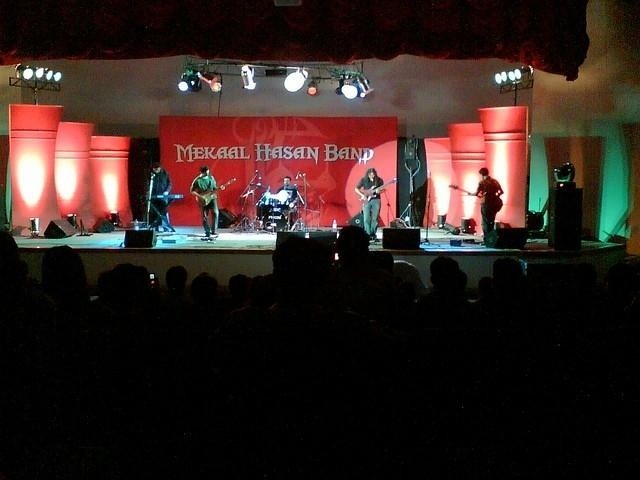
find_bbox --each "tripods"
[396,161,422,227]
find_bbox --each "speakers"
[92,217,113,233]
[43,219,76,238]
[549,187,583,251]
[484,227,529,249]
[382,227,421,250]
[218,208,238,229]
[349,212,364,229]
[125,229,157,248]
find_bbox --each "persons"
[0,221,640,480]
[141,162,173,234]
[189,165,225,241]
[474,168,504,247]
[274,175,299,232]
[353,168,384,242]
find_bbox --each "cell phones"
[149,273,155,285]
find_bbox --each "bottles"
[134,218,139,231]
[332,220,337,234]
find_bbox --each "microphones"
[296,171,299,180]
[151,173,155,178]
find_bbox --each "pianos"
[145,194,184,200]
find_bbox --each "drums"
[277,199,289,207]
[263,212,287,233]
[256,203,273,221]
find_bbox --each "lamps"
[8,63,64,93]
[494,65,535,94]
[493,221,500,230]
[176,57,372,102]
[110,210,121,229]
[30,217,40,239]
[67,212,77,232]
[437,213,446,229]
[462,217,471,234]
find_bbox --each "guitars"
[361,179,397,201]
[196,178,236,205]
[449,185,482,198]
